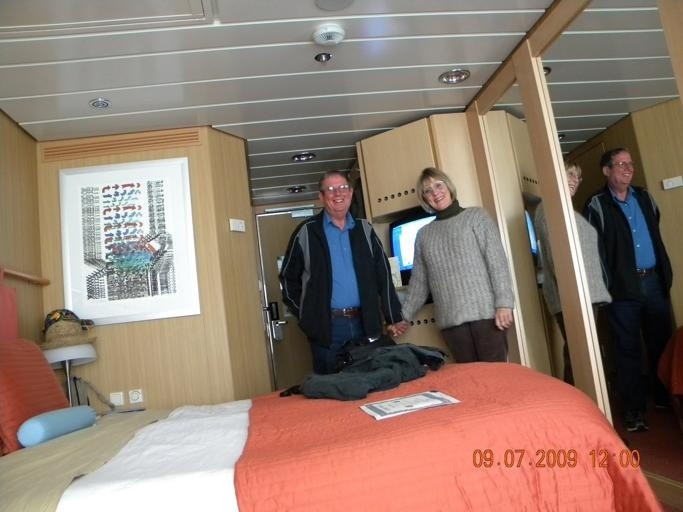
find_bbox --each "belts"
[638,267,657,275]
[330,308,360,317]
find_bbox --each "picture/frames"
[57,157,201,329]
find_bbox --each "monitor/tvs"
[387,212,436,287]
[524,206,540,254]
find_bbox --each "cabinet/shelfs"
[356,112,482,225]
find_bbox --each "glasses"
[609,161,632,169]
[321,184,350,193]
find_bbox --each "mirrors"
[481,2,681,511]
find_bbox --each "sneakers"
[626,416,648,433]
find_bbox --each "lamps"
[44,343,98,406]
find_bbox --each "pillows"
[1,338,96,461]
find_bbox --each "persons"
[582,148,676,432]
[279,170,409,375]
[393,168,515,363]
[533,159,612,386]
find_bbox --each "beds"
[1,359,621,512]
